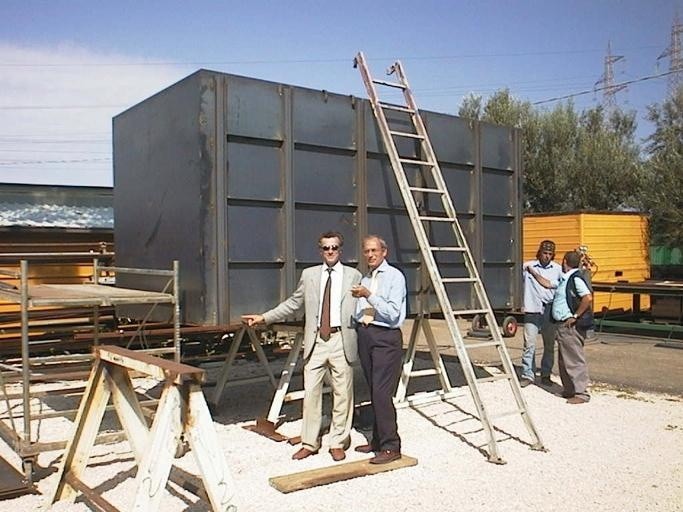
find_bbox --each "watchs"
[573,313,580,319]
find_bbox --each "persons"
[348,234,407,464]
[519,239,563,388]
[240,228,363,460]
[522,251,595,404]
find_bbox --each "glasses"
[363,248,385,256]
[323,245,338,250]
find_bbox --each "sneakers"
[521,379,531,387]
[542,376,553,386]
[567,398,585,404]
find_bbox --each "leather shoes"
[292,448,318,459]
[329,449,346,461]
[355,445,380,453]
[369,450,401,464]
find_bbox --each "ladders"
[353,53,546,465]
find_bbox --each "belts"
[331,326,341,333]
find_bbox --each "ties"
[363,270,378,325]
[320,268,333,342]
